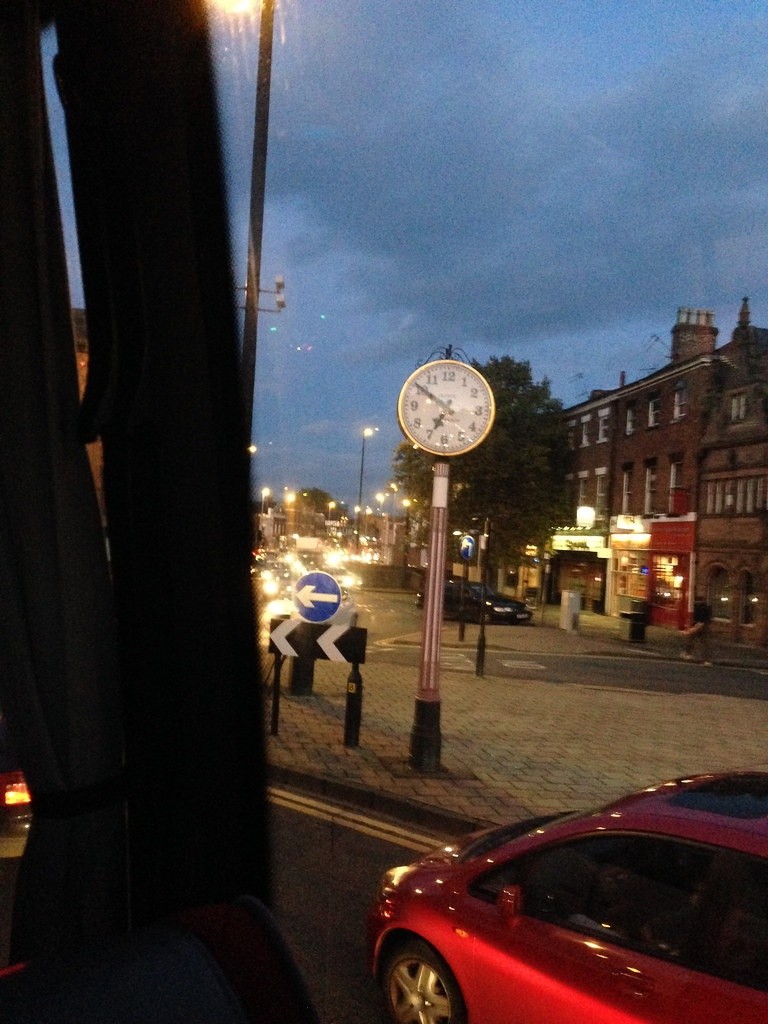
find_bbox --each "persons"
[678,597,713,666]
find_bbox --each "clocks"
[396,359,496,461]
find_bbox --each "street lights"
[353,426,376,554]
[375,492,386,542]
[390,483,399,549]
[365,505,373,541]
[259,486,270,549]
[327,501,337,540]
[403,497,412,589]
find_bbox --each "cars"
[372,766,768,1024]
[415,579,535,624]
[0,753,32,866]
[251,536,381,600]
